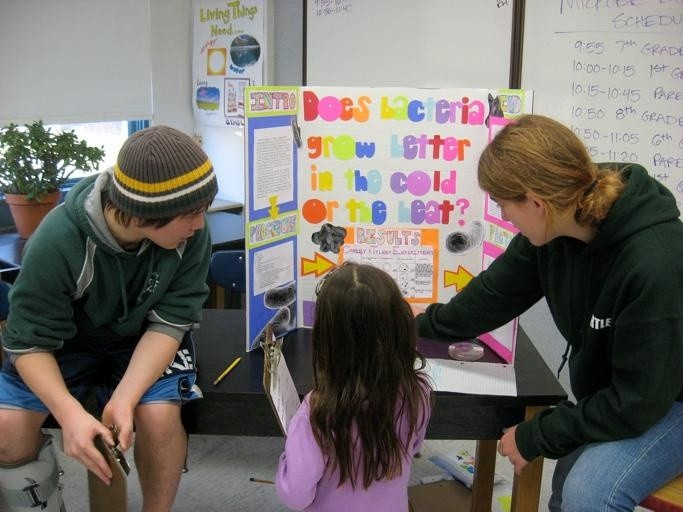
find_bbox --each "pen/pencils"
[250,477,273,483]
[212,357,241,387]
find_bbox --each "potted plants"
[0,119,106,241]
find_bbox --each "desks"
[0,196,571,512]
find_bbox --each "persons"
[0,126,217,512]
[414,114,683,512]
[275,263,439,512]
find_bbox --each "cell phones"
[103,430,132,478]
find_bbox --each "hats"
[108,124,218,219]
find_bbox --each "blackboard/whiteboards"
[304,1,513,89]
[517,2,682,219]
